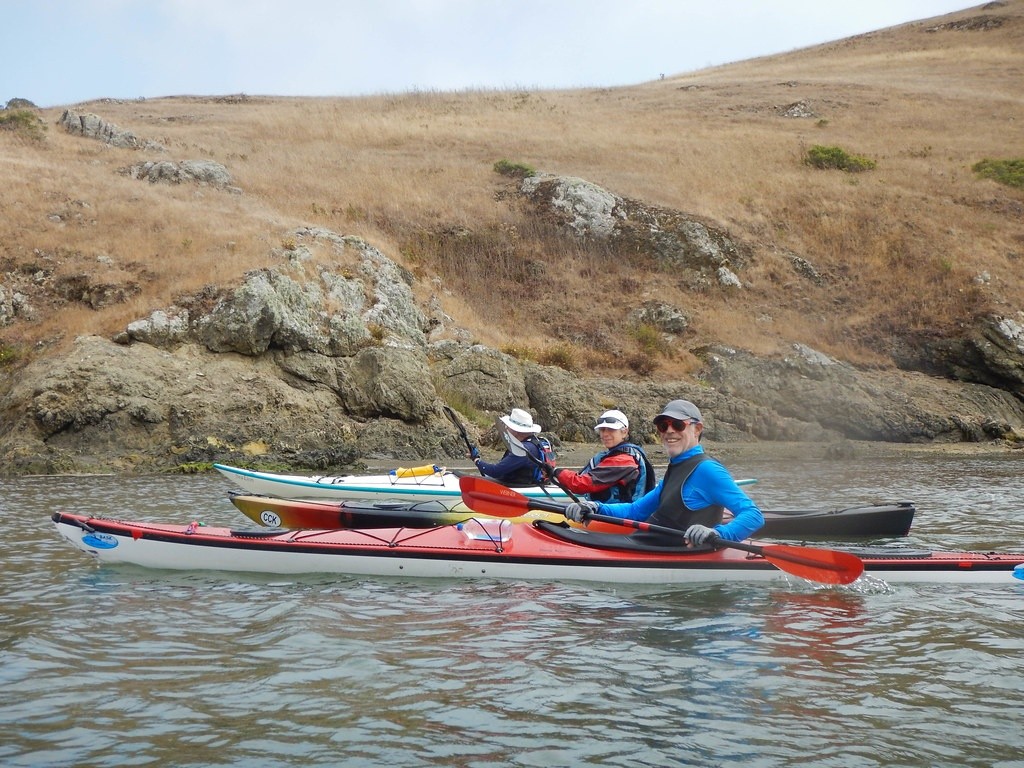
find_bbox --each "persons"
[465,409,546,488]
[565,398,765,550]
[541,410,655,504]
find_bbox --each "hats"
[653,400,703,436]
[594,410,628,430]
[497,408,542,433]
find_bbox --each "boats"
[213,461,759,504]
[49,509,1024,584]
[227,488,918,540]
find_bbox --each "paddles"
[494,414,580,503]
[442,405,485,477]
[459,473,863,586]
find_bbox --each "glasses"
[657,420,698,433]
[597,417,627,427]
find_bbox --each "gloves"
[471,446,480,461]
[544,462,562,478]
[565,500,598,527]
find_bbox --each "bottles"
[457,517,513,542]
